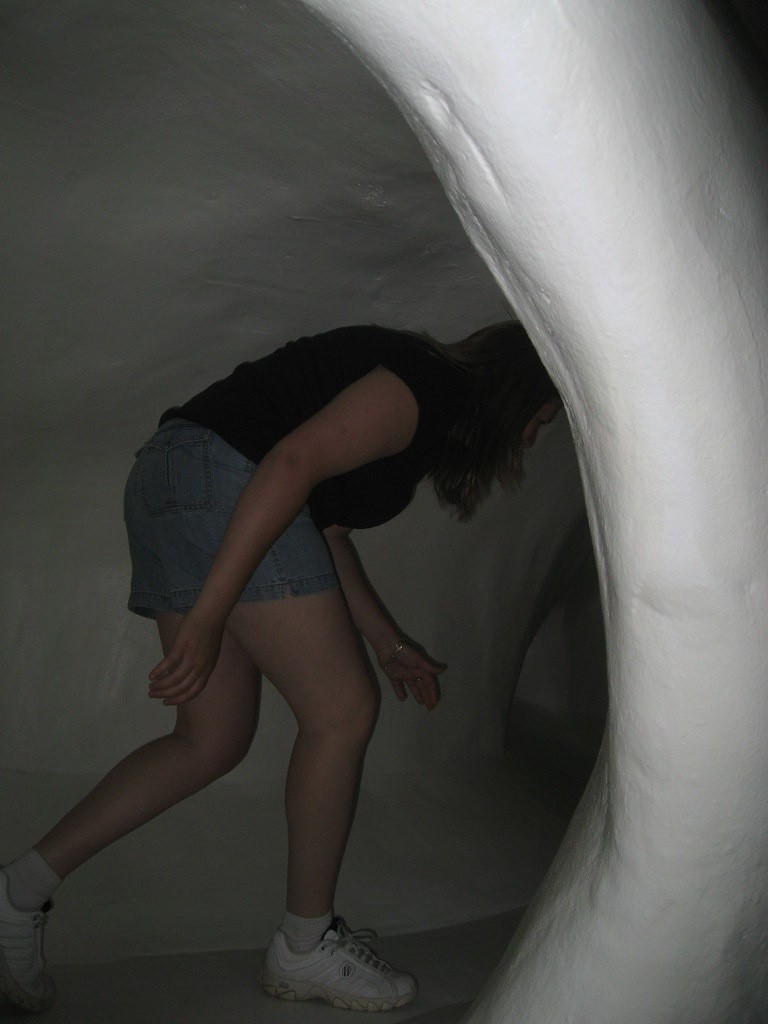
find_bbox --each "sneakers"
[0,863,55,1013]
[257,915,418,1011]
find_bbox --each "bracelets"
[382,636,411,675]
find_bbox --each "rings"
[412,677,421,683]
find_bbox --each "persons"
[0,319,566,1014]
